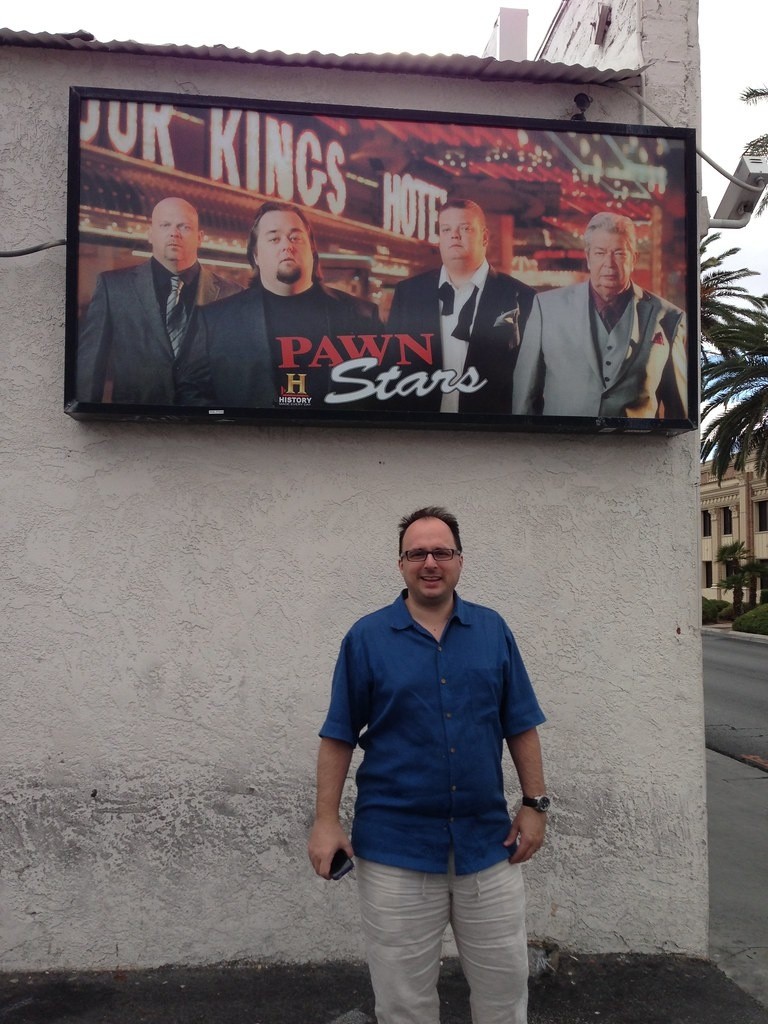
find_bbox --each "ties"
[602,306,613,335]
[166,276,187,356]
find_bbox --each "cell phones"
[329,850,354,880]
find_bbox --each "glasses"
[400,547,461,562]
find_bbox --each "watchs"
[521,795,551,812]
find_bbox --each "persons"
[75,194,688,420]
[307,505,547,1024]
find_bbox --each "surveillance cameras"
[713,155,768,221]
[574,93,593,111]
